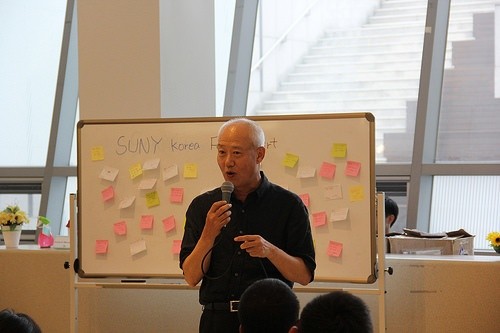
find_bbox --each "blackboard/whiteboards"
[77,111,376,284]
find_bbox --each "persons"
[385,196,399,236]
[299,291,372,333]
[179,117,316,333]
[238,278,300,333]
[0,308,42,332]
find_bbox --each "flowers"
[0,205,29,232]
[485,231,500,250]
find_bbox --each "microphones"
[221,182,234,230]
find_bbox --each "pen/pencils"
[121,280,146,283]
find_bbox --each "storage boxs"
[387,227,476,256]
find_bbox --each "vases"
[493,245,500,253]
[1,225,23,249]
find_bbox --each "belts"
[205,299,240,312]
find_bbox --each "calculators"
[403,228,445,238]
